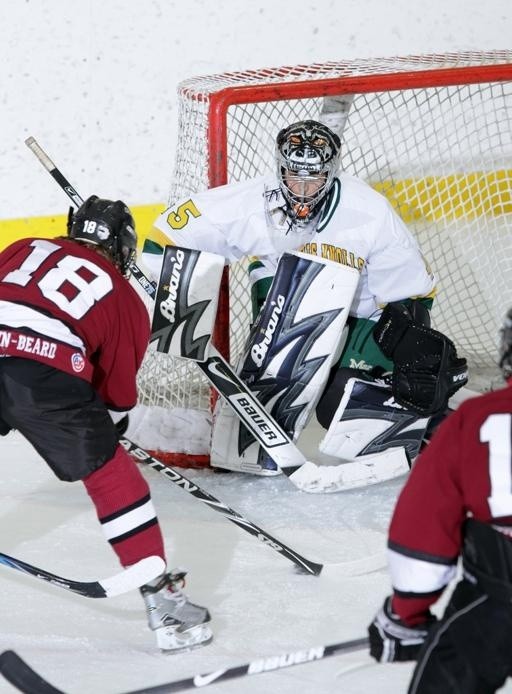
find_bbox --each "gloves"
[367,597,437,664]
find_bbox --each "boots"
[141,571,210,637]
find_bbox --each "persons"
[362,309,512,694]
[145,119,455,476]
[0,194,214,631]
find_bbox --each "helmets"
[66,194,137,281]
[497,308,511,378]
[275,121,342,236]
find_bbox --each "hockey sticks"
[120,438,388,576]
[2,637,372,694]
[25,136,412,493]
[0,554,165,599]
